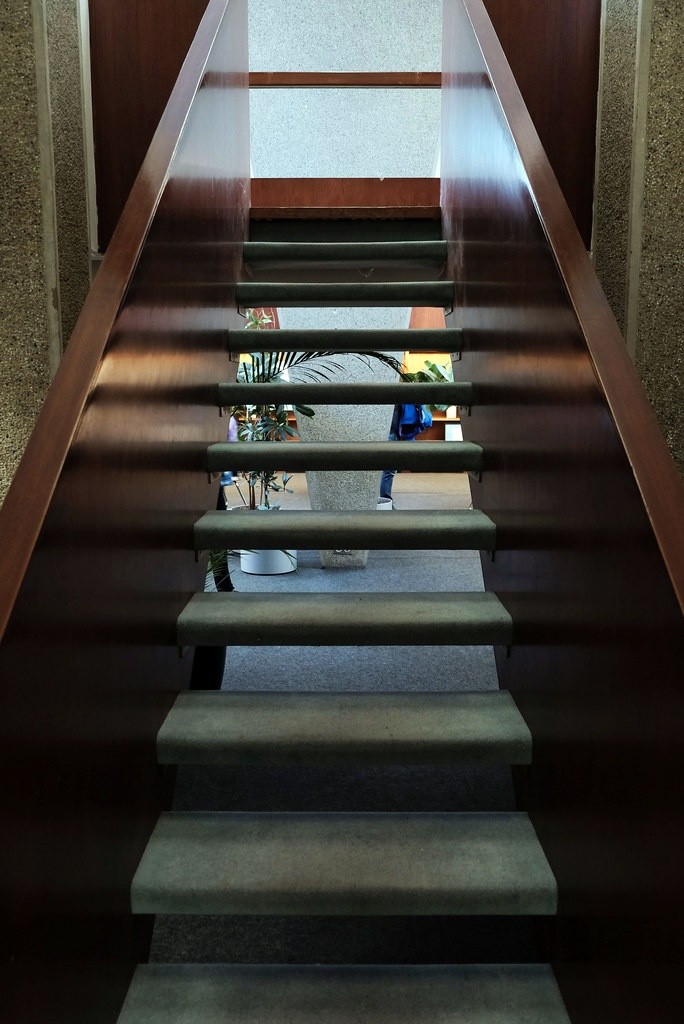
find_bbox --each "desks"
[238,417,462,425]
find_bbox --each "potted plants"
[231,307,456,574]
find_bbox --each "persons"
[380,405,400,498]
[210,486,238,593]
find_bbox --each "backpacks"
[399,404,424,439]
